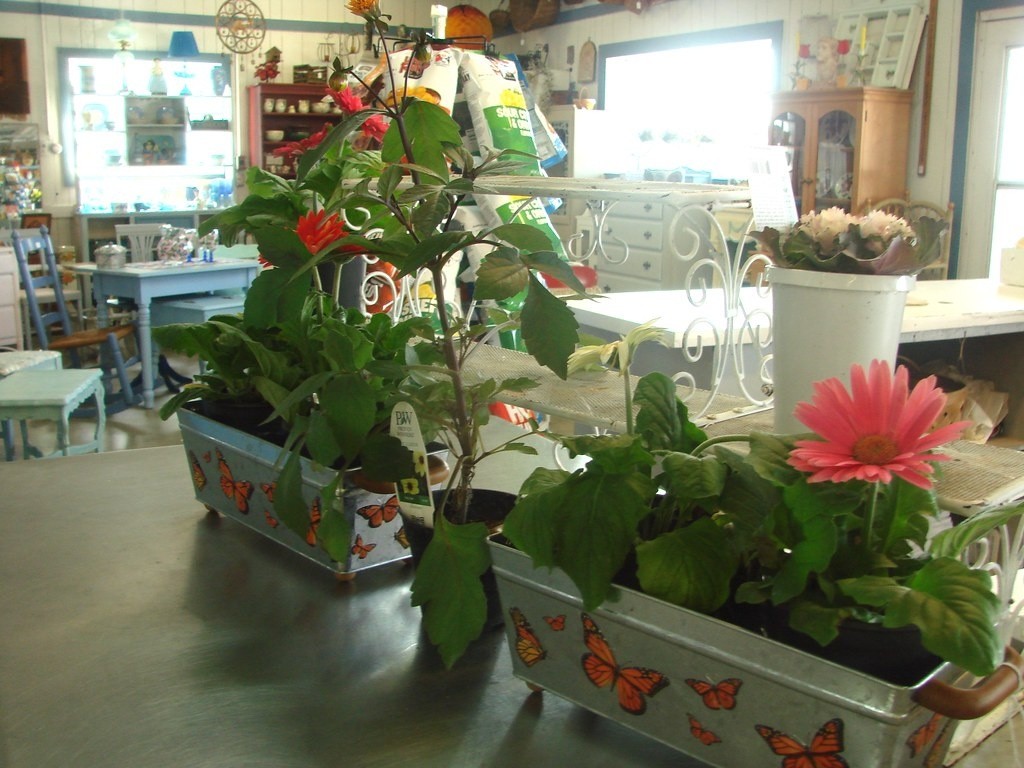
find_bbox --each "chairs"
[115,225,171,263]
[11,224,192,417]
[12,231,83,351]
[312,253,367,329]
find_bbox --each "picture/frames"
[19,214,51,263]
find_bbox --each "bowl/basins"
[265,131,285,142]
[289,124,311,142]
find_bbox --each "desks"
[86,257,259,408]
[4,417,1024,768]
[568,278,1022,388]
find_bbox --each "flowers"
[0,166,40,219]
[153,0,1022,678]
[752,195,948,275]
[186,184,231,203]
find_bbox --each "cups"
[263,98,343,113]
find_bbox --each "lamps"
[112,20,135,74]
[168,32,199,60]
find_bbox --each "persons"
[816,37,839,71]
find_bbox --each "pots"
[92,241,128,268]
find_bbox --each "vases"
[765,264,917,437]
[178,390,449,580]
[485,525,1024,767]
[397,488,522,634]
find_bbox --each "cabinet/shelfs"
[712,206,755,288]
[1,246,23,353]
[247,83,343,180]
[73,215,215,307]
[540,104,592,264]
[764,89,912,225]
[590,197,712,294]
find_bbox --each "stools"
[0,369,105,456]
[0,350,63,461]
